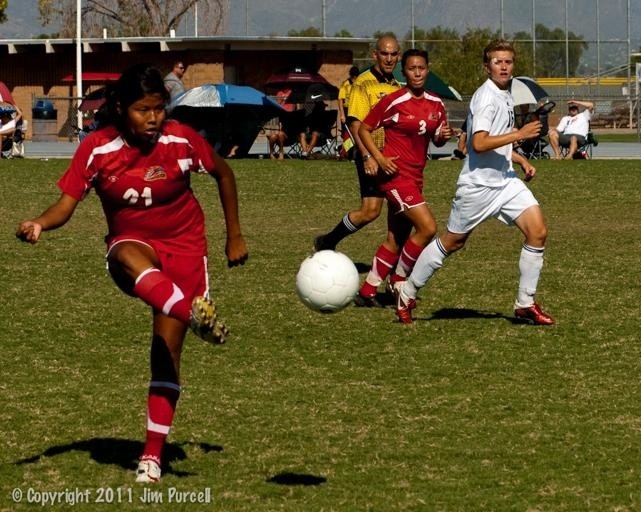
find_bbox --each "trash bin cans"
[32,99,58,142]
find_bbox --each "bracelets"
[373,149,380,157]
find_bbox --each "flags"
[343,137,355,152]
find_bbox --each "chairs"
[264,109,339,160]
[1,106,24,157]
[516,113,595,160]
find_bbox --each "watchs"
[362,153,372,162]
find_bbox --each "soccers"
[296,250,360,312]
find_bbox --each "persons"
[17,67,250,485]
[511,104,541,158]
[314,36,401,254]
[452,118,469,161]
[354,49,453,310]
[339,67,362,161]
[393,41,557,324]
[1,102,22,158]
[550,98,596,162]
[164,62,186,99]
[270,88,336,162]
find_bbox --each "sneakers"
[314,236,336,251]
[135,461,162,484]
[359,273,418,324]
[513,299,556,326]
[190,297,229,344]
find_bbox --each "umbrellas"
[356,60,464,104]
[165,83,286,158]
[506,76,551,127]
[78,83,118,113]
[265,72,331,112]
